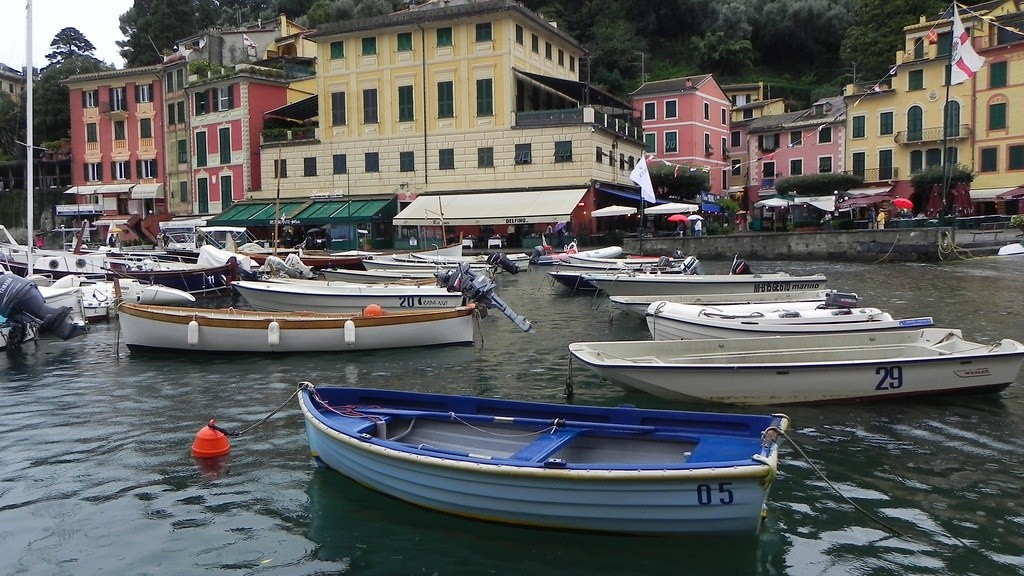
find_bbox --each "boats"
[294,378,788,540]
[607,288,863,327]
[566,320,1019,409]
[583,254,833,300]
[111,276,485,354]
[231,258,543,337]
[652,296,938,340]
[0,1,827,346]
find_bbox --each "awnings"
[264,94,318,122]
[846,185,896,197]
[835,196,892,211]
[514,67,636,115]
[210,196,395,224]
[63,184,164,200]
[158,215,217,230]
[969,186,1024,202]
[393,188,588,226]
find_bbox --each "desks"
[461,239,473,249]
[487,239,501,248]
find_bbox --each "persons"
[867,207,885,230]
[676,219,687,237]
[691,219,702,236]
[824,211,832,222]
[109,233,121,247]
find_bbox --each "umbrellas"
[643,202,699,214]
[752,194,793,232]
[667,214,703,225]
[927,181,974,216]
[894,198,913,208]
[590,204,638,229]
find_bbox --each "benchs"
[507,432,581,463]
[969,231,1004,243]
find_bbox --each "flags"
[597,183,669,204]
[629,152,656,203]
[949,3,987,85]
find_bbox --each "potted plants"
[362,238,373,250]
[1009,214,1024,232]
